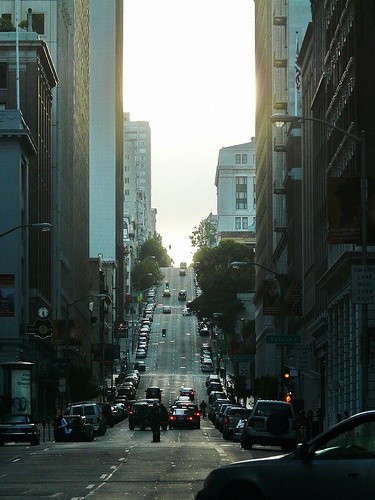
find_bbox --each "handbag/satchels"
[65,427,72,434]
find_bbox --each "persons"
[335,411,350,424]
[148,403,168,443]
[56,415,72,441]
[199,400,207,418]
[11,397,27,413]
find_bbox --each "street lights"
[269,112,372,413]
[228,260,287,401]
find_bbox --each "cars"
[194,408,375,500]
[177,387,199,401]
[180,261,188,269]
[101,279,251,443]
[144,387,163,401]
[179,269,186,276]
[0,413,41,446]
[170,408,194,430]
[51,414,95,442]
[129,403,161,432]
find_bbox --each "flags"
[134,292,143,303]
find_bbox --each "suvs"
[68,403,107,436]
[240,399,299,453]
